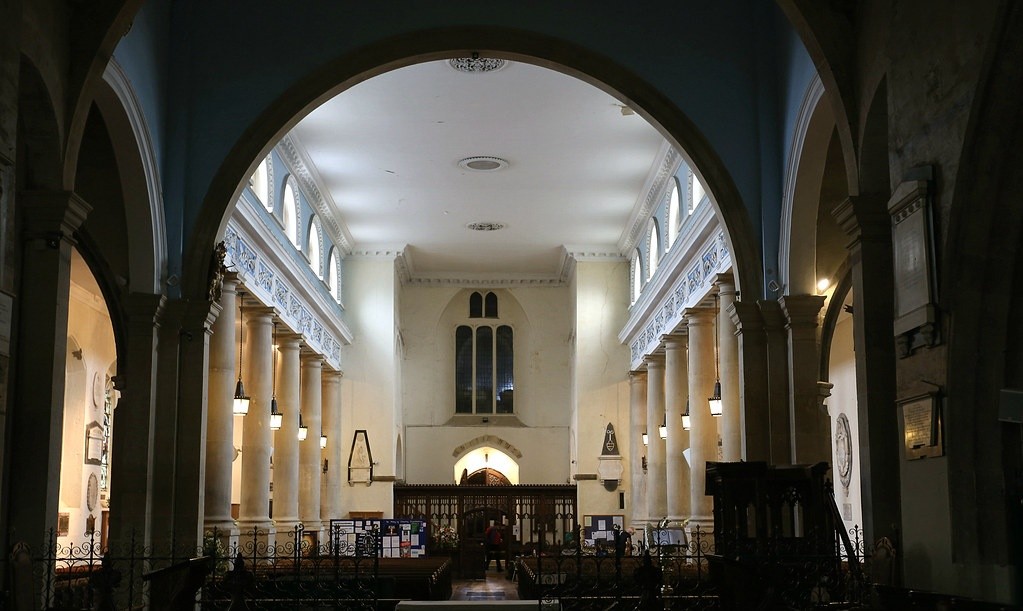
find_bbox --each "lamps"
[707,294,723,417]
[298,345,308,440]
[680,323,691,431]
[320,363,328,450]
[658,413,666,440]
[642,426,649,446]
[233,292,251,417]
[270,322,284,431]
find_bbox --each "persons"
[619,527,637,556]
[835,418,848,472]
[484,521,505,573]
[224,552,245,571]
